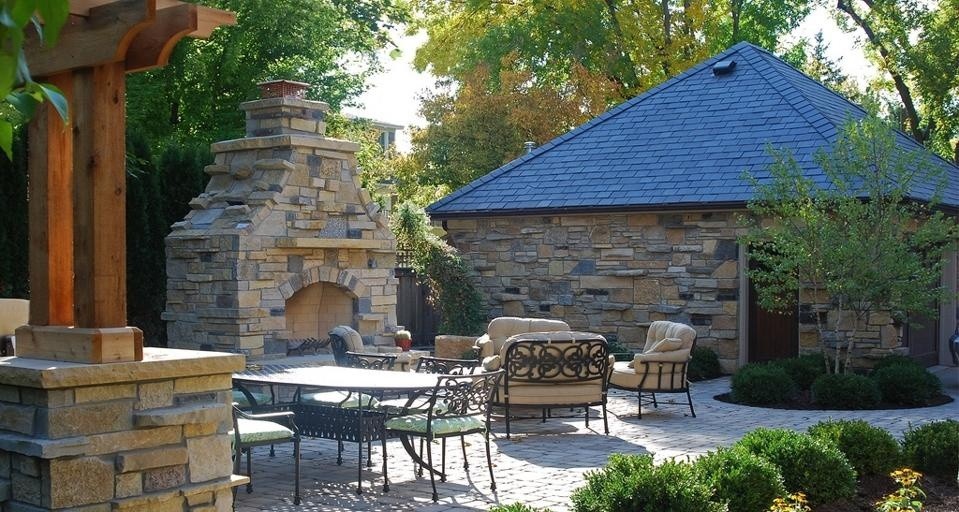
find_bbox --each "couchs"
[474,316,696,439]
[330,327,411,370]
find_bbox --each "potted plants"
[395,330,412,352]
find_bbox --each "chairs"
[231,386,279,461]
[298,350,397,468]
[365,355,480,479]
[231,402,302,507]
[380,366,505,503]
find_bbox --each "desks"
[232,363,471,494]
